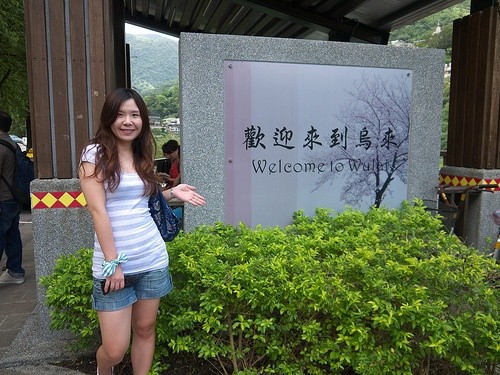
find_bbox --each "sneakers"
[0,268,25,284]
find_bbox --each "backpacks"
[0,140,36,211]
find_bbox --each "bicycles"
[417,182,500,268]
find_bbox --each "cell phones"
[100,279,111,295]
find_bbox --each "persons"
[155,140,181,190]
[0,111,25,283]
[79,88,206,375]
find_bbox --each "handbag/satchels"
[148,183,183,243]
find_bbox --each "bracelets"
[101,253,128,277]
[171,187,174,198]
